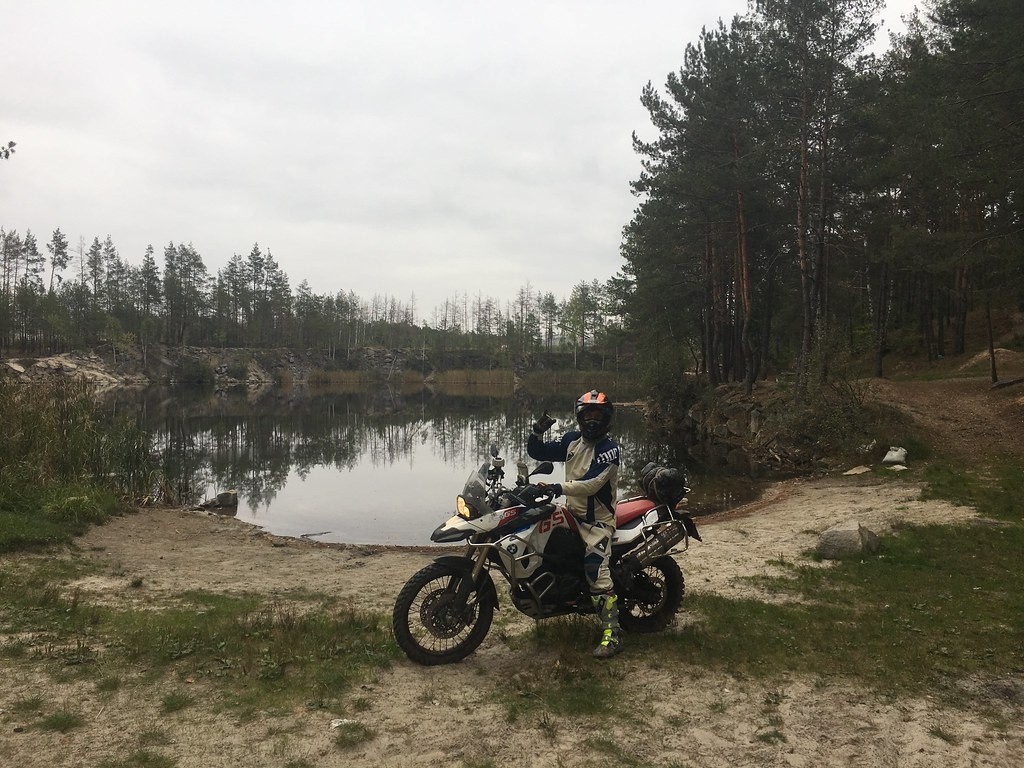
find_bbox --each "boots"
[592,595,624,657]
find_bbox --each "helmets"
[575,389,614,441]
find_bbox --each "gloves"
[533,410,557,436]
[538,482,562,499]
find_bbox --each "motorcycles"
[392,445,703,667]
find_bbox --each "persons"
[528,389,625,659]
